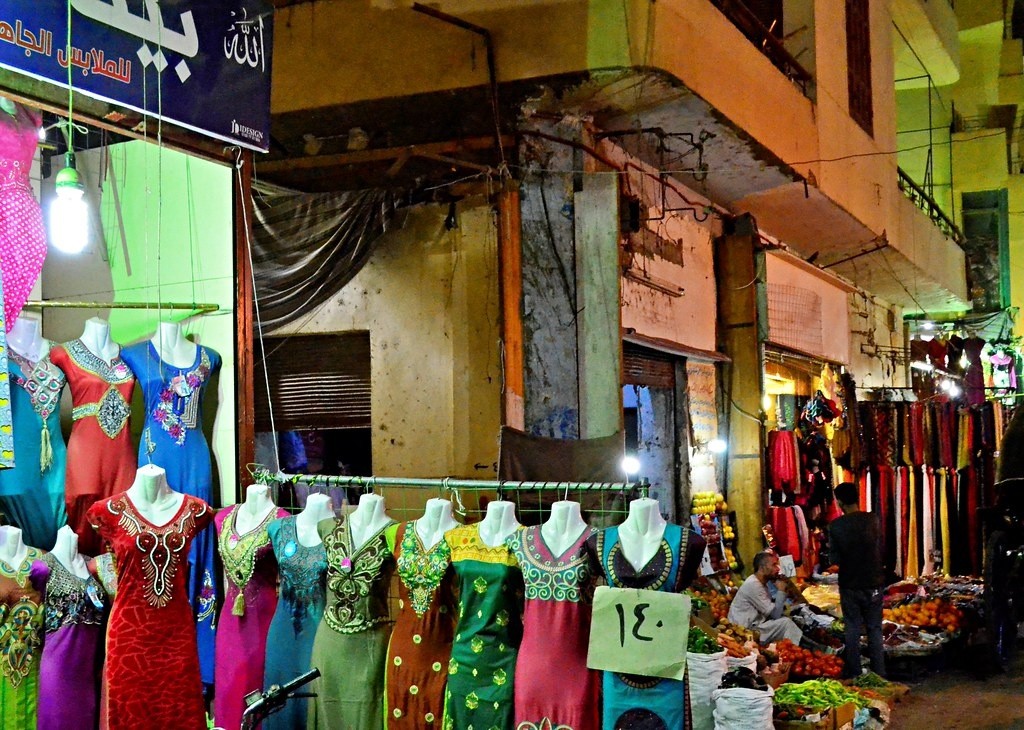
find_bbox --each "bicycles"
[975,506,1024,673]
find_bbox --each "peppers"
[773,679,872,712]
[677,590,724,654]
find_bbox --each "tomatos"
[685,587,733,620]
[773,637,844,678]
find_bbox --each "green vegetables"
[847,670,895,689]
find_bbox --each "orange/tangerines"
[882,598,964,632]
[692,492,727,515]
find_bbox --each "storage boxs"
[772,704,855,729]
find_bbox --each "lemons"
[722,520,739,569]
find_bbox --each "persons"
[0,463,706,730]
[726,554,843,652]
[828,482,887,683]
[0,97,221,685]
[910,331,1017,406]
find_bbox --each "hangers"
[869,385,1008,406]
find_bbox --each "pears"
[715,618,761,644]
